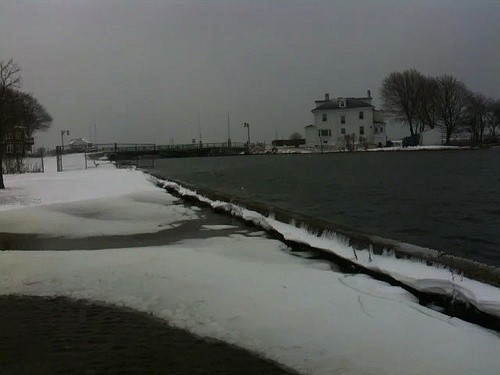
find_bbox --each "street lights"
[242,122,250,148]
[59,130,70,153]
